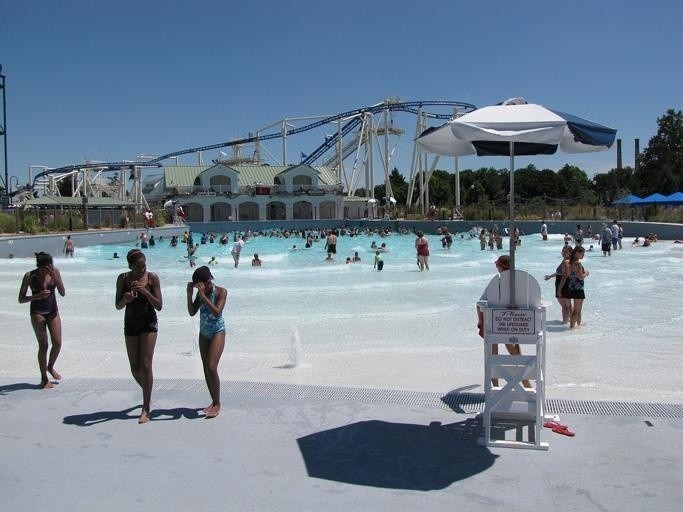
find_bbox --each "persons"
[478,227,521,250]
[185,265,228,419]
[114,248,162,424]
[476,254,534,392]
[429,207,435,220]
[541,221,547,240]
[43,213,54,227]
[568,245,590,329]
[136,226,474,272]
[551,210,561,220]
[113,253,119,258]
[63,235,74,257]
[176,207,184,221]
[143,208,154,229]
[543,243,573,324]
[380,207,385,218]
[565,220,682,257]
[17,250,65,389]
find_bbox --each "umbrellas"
[660,192,683,220]
[638,193,667,215]
[610,193,643,222]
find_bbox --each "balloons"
[413,94,619,308]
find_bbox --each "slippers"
[543,421,575,436]
